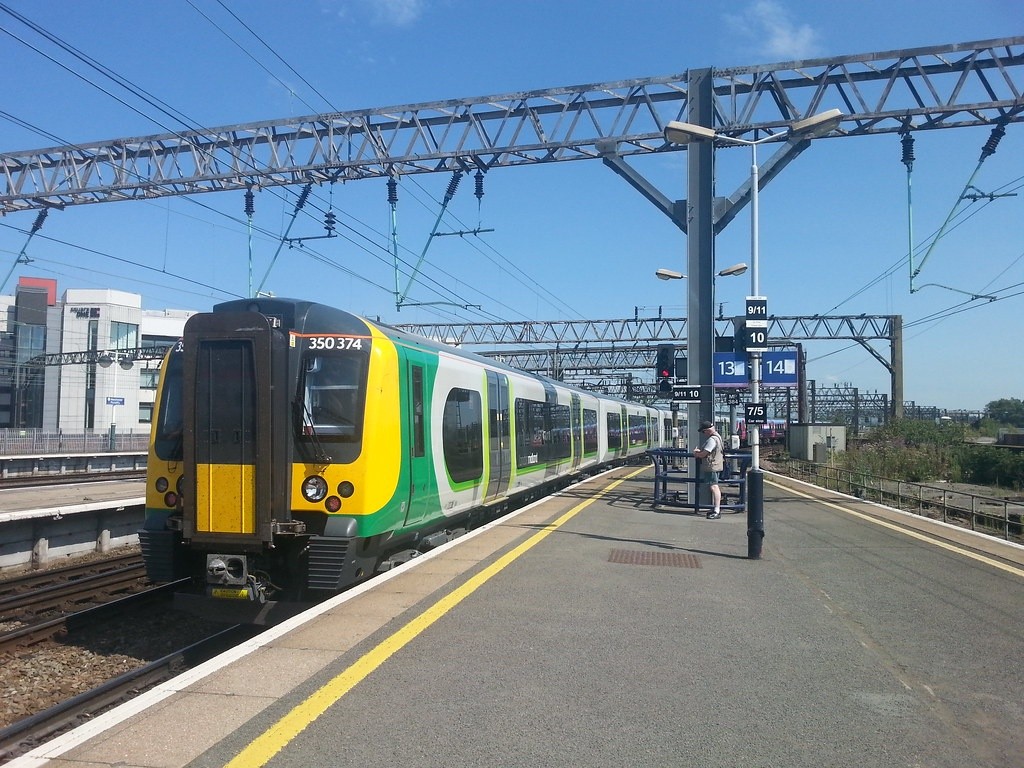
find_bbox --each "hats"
[698,421,713,432]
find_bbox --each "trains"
[136,298,832,627]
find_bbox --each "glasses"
[702,430,709,433]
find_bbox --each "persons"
[694,421,725,519]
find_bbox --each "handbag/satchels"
[719,461,731,480]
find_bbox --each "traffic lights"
[656,343,675,378]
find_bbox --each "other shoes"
[707,510,721,519]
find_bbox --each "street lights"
[664,107,846,560]
[98,350,134,451]
